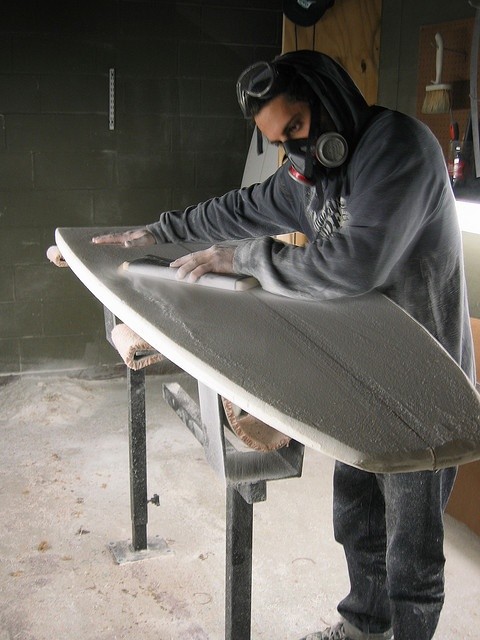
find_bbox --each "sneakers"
[299,621,391,639]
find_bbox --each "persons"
[92,50,478,640]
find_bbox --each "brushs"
[421,31,452,114]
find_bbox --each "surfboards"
[54,227,479,475]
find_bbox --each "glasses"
[236,61,274,120]
